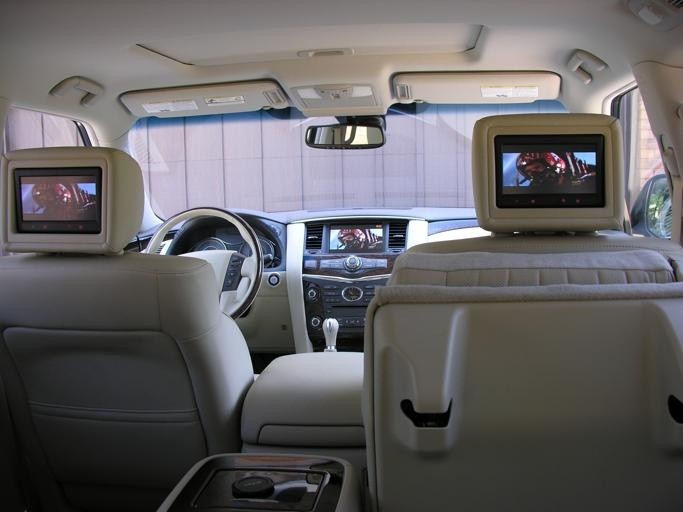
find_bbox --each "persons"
[516,151,596,187]
[32,183,96,216]
[338,229,383,250]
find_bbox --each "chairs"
[0,149,258,512]
[361,112,683,512]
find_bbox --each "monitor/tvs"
[14,167,102,234]
[494,134,605,209]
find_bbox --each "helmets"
[337,229,366,242]
[31,182,72,211]
[515,151,566,181]
